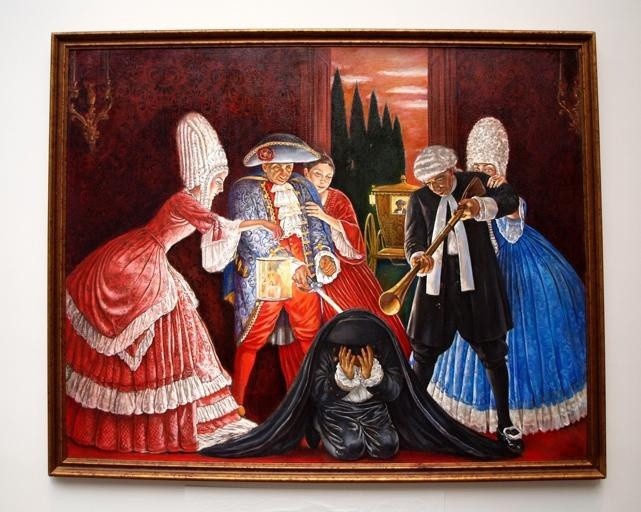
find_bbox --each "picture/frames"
[48,29,606,483]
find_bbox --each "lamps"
[369,192,376,207]
[256,240,292,302]
